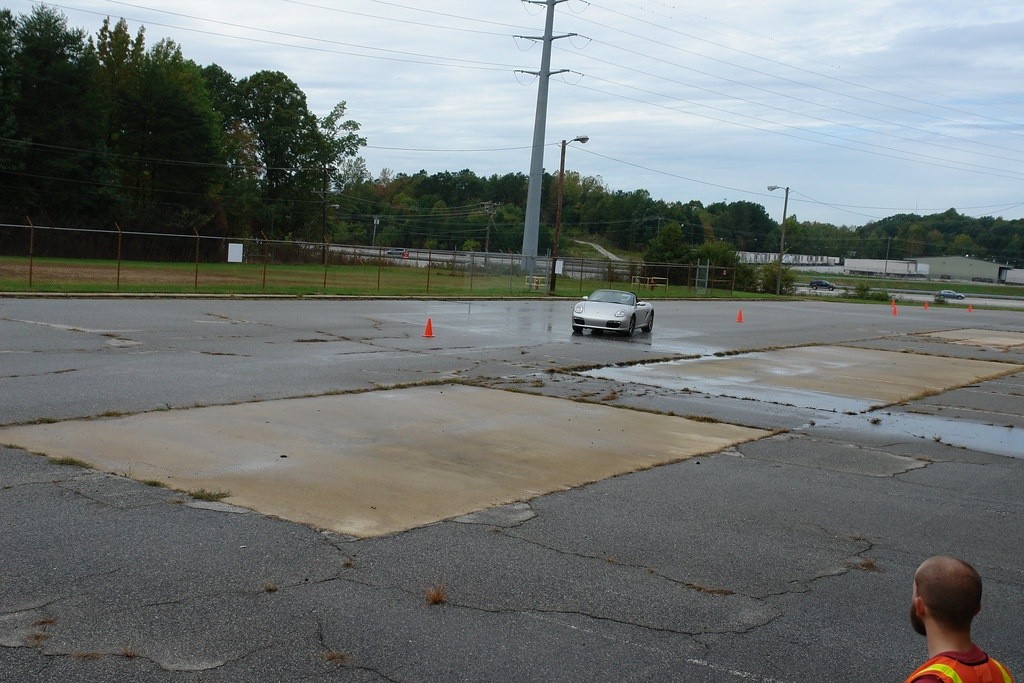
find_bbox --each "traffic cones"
[924,302,928,310]
[968,304,972,312]
[891,299,895,307]
[736,310,743,322]
[893,307,897,315]
[422,317,435,337]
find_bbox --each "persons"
[905,555,1014,683]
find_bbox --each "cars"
[936,290,965,300]
[572,289,654,336]
[809,280,837,291]
[387,248,406,255]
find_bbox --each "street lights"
[767,185,789,295]
[322,205,339,264]
[550,135,589,291]
